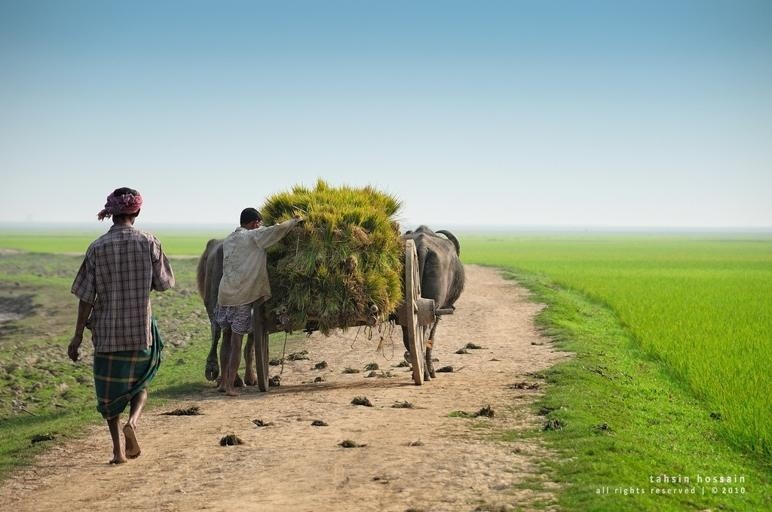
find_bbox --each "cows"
[196,239,258,388]
[396,225,465,382]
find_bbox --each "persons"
[216,207,304,397]
[67,187,176,464]
